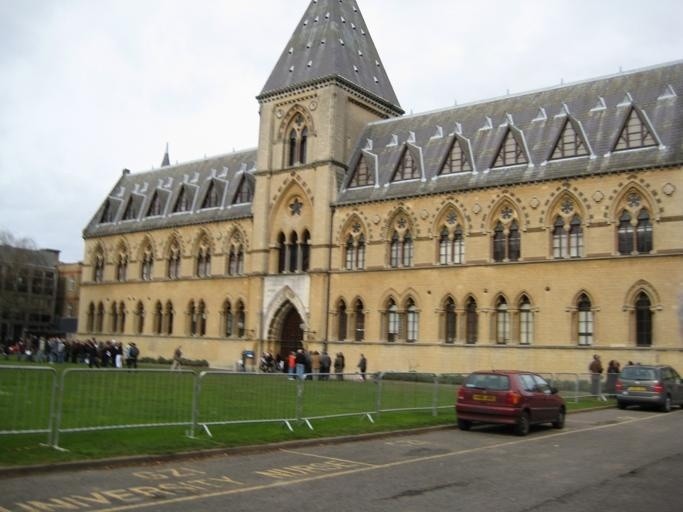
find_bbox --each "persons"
[588,355,633,398]
[259,347,346,381]
[170,344,183,373]
[357,353,367,381]
[0,336,139,368]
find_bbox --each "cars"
[615,364,683,412]
[454,369,567,435]
